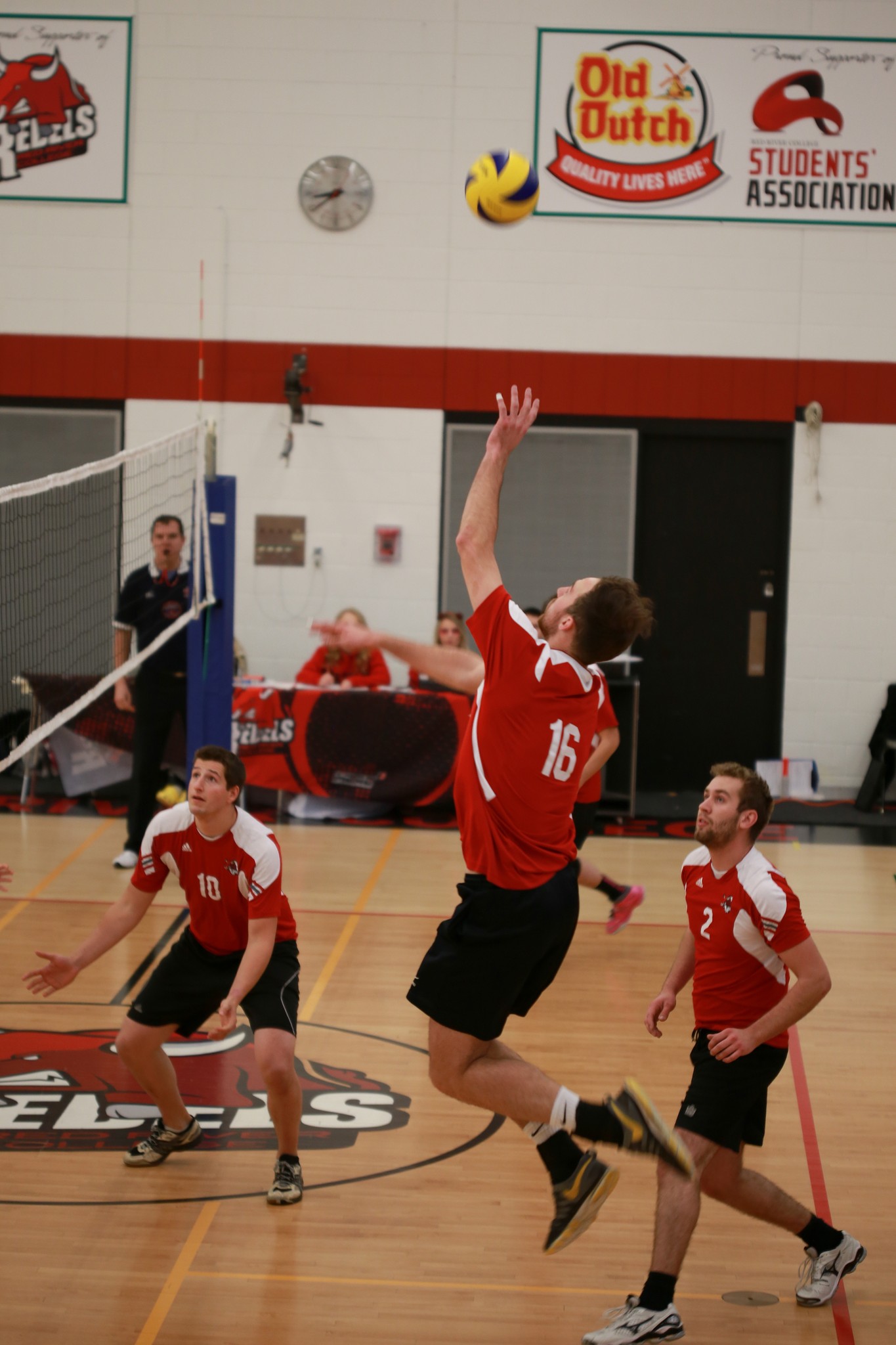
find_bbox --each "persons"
[23,745,304,1205]
[111,515,194,870]
[294,606,391,690]
[581,762,866,1345]
[407,384,696,1252]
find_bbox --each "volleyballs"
[466,149,539,225]
[156,784,187,806]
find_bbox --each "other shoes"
[113,848,139,870]
[605,884,644,931]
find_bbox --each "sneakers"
[267,1153,304,1206]
[794,1228,868,1306]
[582,1292,685,1345]
[601,1077,694,1185]
[541,1147,620,1257]
[121,1114,204,1166]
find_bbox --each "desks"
[9,669,479,822]
